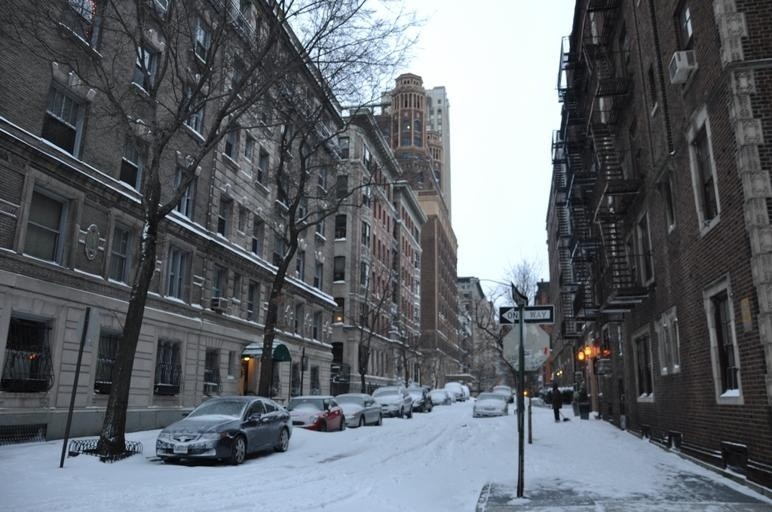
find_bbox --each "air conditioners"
[666,46,700,86]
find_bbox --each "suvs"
[406,387,432,413]
[372,387,413,419]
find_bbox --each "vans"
[445,382,465,402]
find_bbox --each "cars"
[472,392,508,417]
[334,393,382,429]
[155,396,292,464]
[285,396,346,433]
[428,390,453,406]
[492,385,515,403]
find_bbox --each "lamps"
[573,339,613,363]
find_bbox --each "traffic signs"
[499,304,555,326]
[511,282,529,307]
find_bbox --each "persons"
[551,382,562,421]
